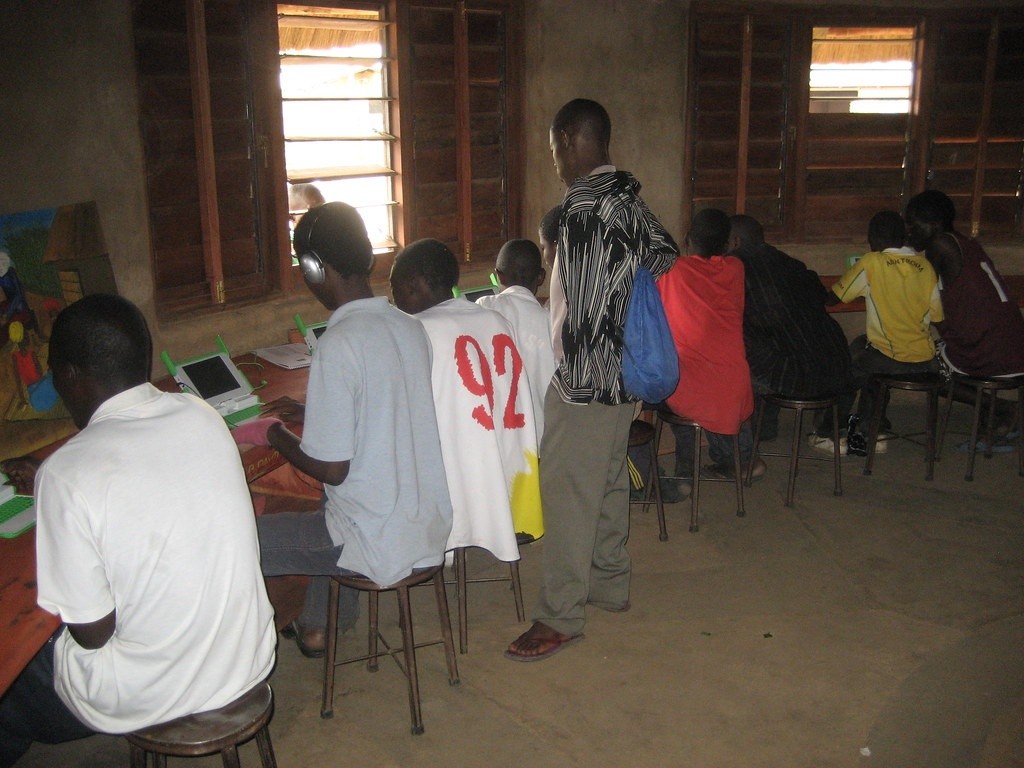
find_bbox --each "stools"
[935,372,1024,482]
[626,419,668,542]
[858,373,940,482]
[320,561,459,736]
[744,393,844,508]
[642,411,746,534]
[126,680,277,768]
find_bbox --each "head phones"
[300,206,327,285]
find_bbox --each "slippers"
[955,440,1017,454]
[992,428,1021,445]
[702,464,762,483]
[504,621,585,662]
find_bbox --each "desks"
[0,272,1023,701]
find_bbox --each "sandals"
[280,622,326,659]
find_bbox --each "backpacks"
[622,266,680,405]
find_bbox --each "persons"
[0,289,279,768]
[537,204,565,268]
[907,187,1023,440]
[506,97,682,663]
[229,199,452,660]
[807,210,944,456]
[474,238,557,463]
[654,208,770,484]
[388,238,543,562]
[723,213,853,453]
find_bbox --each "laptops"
[452,272,501,305]
[294,315,328,355]
[0,471,37,538]
[159,334,272,429]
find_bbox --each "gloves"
[231,418,284,446]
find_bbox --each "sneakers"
[847,432,887,453]
[808,434,849,457]
